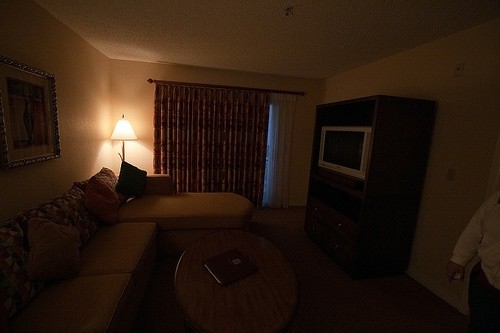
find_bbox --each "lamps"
[111,114,138,160]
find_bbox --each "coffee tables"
[173,229,300,333]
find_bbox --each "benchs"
[75,173,254,249]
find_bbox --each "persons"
[447,191,500,333]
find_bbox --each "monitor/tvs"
[317,127,371,182]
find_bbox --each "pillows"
[116,161,147,198]
[81,176,124,227]
[27,218,79,250]
[27,222,82,283]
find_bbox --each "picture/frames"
[0,55,62,170]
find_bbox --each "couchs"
[0,187,159,333]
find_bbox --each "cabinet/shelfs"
[304,95,438,273]
